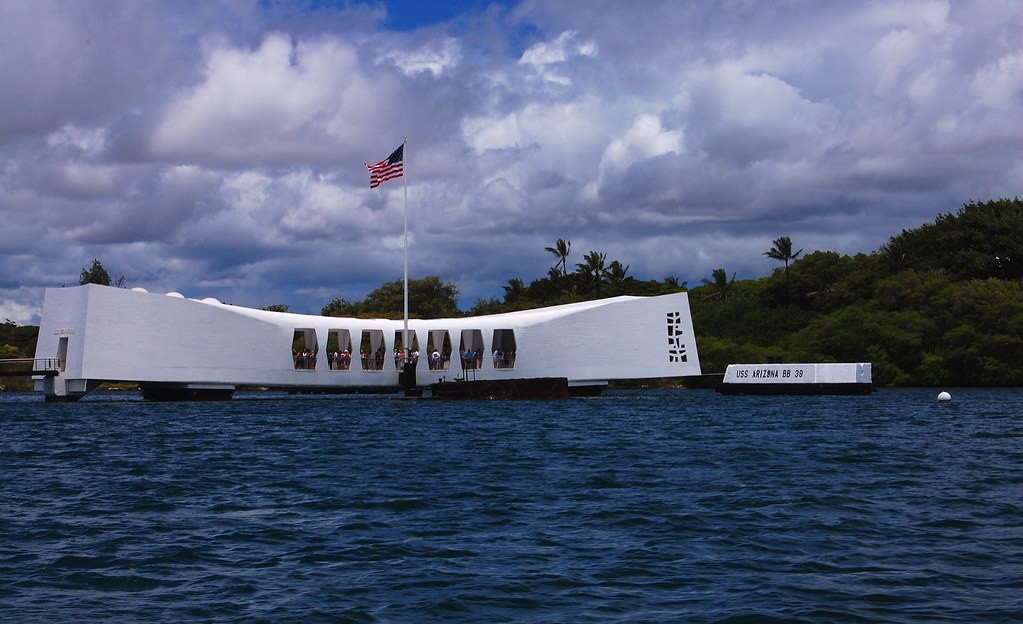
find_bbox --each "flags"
[364,143,405,189]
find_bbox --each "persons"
[360,348,383,371]
[328,350,351,370]
[460,348,482,369]
[292,349,317,370]
[493,348,516,368]
[427,348,450,370]
[394,348,419,370]
